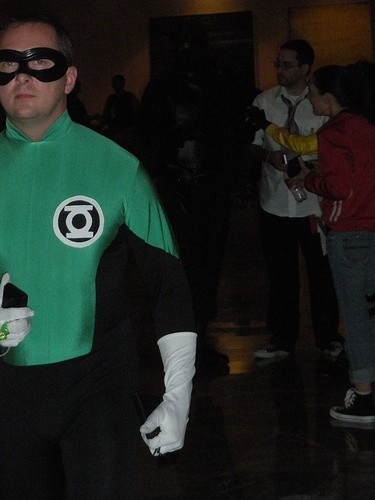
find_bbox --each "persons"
[1,12,198,457]
[247,61,375,154]
[105,74,140,134]
[137,36,259,376]
[67,74,91,128]
[293,65,375,426]
[246,39,345,361]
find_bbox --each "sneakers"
[328,392,375,425]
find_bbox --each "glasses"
[273,61,299,71]
[0,47,72,87]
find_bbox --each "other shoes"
[321,341,342,359]
[253,335,298,358]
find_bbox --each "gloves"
[139,330,198,456]
[244,104,266,135]
[0,271,36,347]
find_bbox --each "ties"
[282,94,307,176]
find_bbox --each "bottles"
[280,154,307,205]
